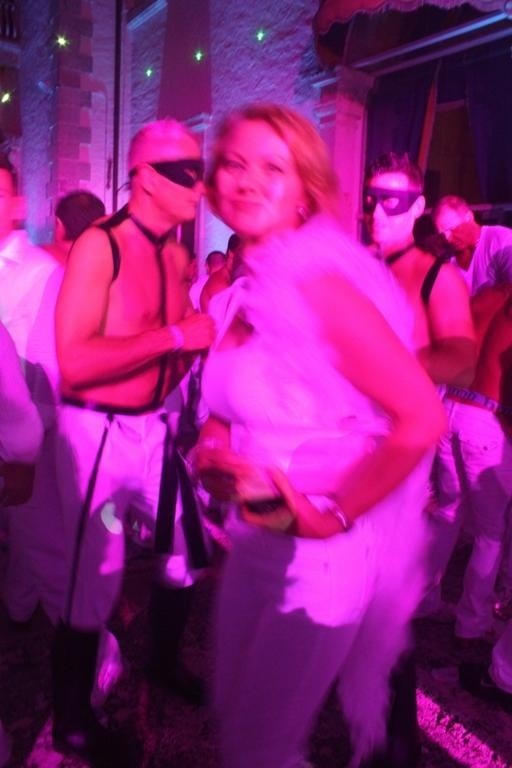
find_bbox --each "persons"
[159,226,248,478]
[1,322,43,464]
[37,116,218,765]
[416,278,512,644]
[433,190,512,299]
[0,149,67,764]
[35,188,106,266]
[359,150,481,765]
[194,99,450,768]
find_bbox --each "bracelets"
[320,494,352,534]
